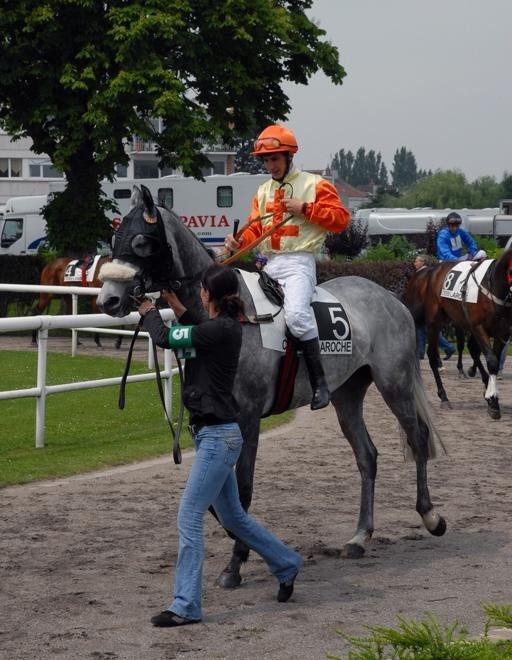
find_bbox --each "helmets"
[249,125,299,156]
[446,212,462,225]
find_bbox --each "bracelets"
[302,203,307,214]
[145,306,156,315]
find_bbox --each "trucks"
[348,200,511,257]
[0,172,334,263]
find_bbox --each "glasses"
[253,137,298,151]
[447,218,462,223]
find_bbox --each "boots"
[299,335,331,411]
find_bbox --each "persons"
[414,254,457,364]
[138,262,302,629]
[213,123,349,412]
[436,210,486,263]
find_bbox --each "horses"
[454,322,488,388]
[403,243,512,421]
[28,256,124,350]
[94,182,449,589]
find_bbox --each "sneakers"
[150,610,203,627]
[275,570,299,602]
[496,368,504,380]
[443,346,456,361]
